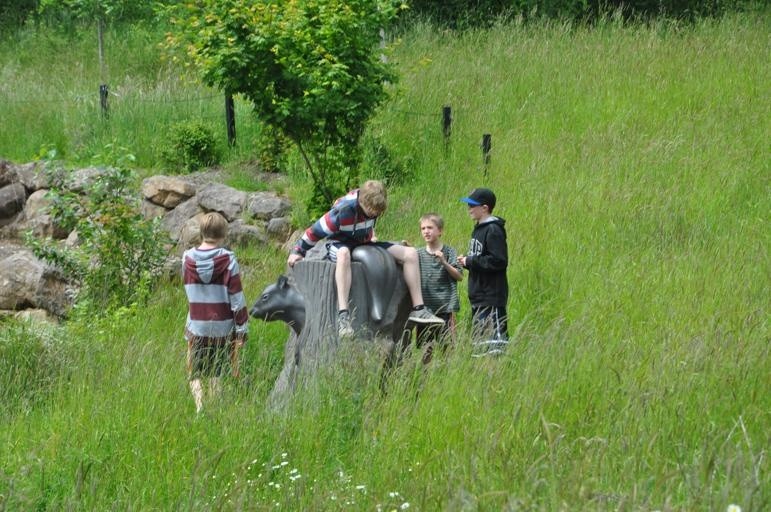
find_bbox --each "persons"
[456,188,511,355]
[182,210,249,422]
[399,212,464,364]
[287,180,442,337]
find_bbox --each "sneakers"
[338,312,354,339]
[408,309,445,327]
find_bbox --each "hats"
[461,188,496,208]
